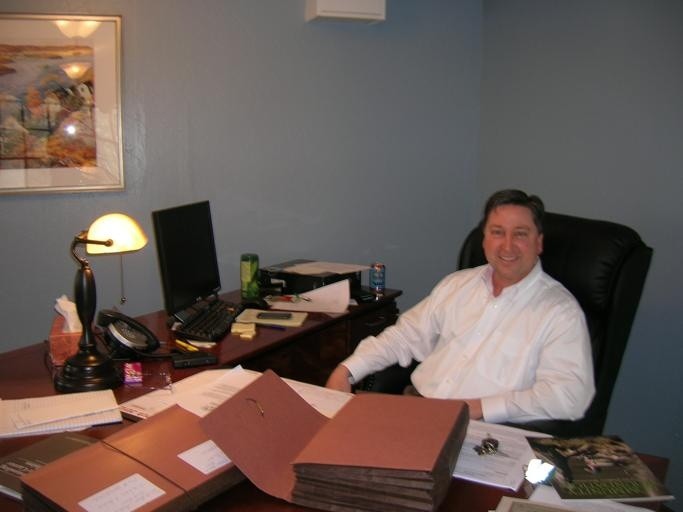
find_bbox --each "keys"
[474,433,511,457]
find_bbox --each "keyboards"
[176,299,244,343]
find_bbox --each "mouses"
[241,297,268,309]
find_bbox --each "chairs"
[350,212,653,437]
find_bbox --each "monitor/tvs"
[152,199,222,325]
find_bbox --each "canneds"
[241,254,259,299]
[368,262,386,292]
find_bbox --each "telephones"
[97,309,160,358]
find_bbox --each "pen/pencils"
[256,323,286,330]
[167,337,217,353]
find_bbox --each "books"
[0,431,100,503]
[526,434,674,501]
[232,306,308,329]
[289,392,470,512]
[454,420,553,492]
[0,390,122,438]
[120,370,353,422]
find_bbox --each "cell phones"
[172,351,217,368]
[257,312,291,320]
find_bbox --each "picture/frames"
[0,12,126,194]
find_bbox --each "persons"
[324,187,597,424]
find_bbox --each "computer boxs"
[258,258,361,300]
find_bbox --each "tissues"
[49,293,86,367]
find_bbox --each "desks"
[0,285,683,512]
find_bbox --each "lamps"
[54,213,149,393]
[59,62,91,80]
[52,21,102,39]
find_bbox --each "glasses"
[487,225,538,242]
